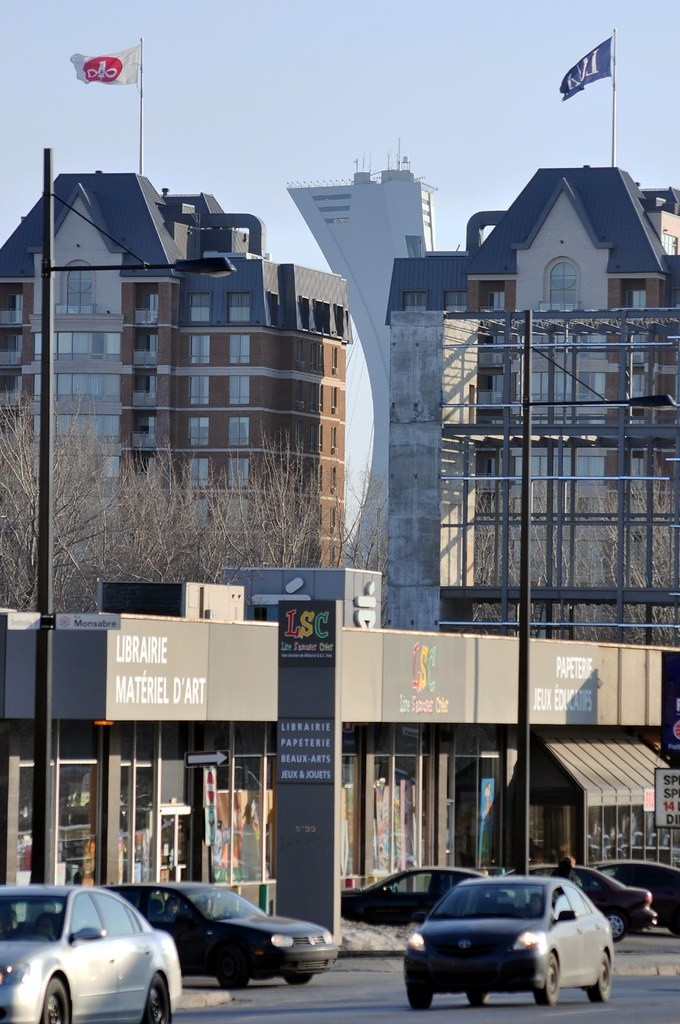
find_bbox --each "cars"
[499,863,660,943]
[577,860,680,937]
[343,865,517,932]
[0,883,183,1024]
[404,874,617,1012]
[98,880,337,989]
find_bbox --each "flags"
[68,45,141,84]
[558,39,614,101]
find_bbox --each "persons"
[73,865,82,884]
[159,897,178,922]
[519,893,543,916]
[194,895,223,920]
[17,912,56,940]
[551,855,582,887]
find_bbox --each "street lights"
[27,148,237,893]
[519,307,679,878]
